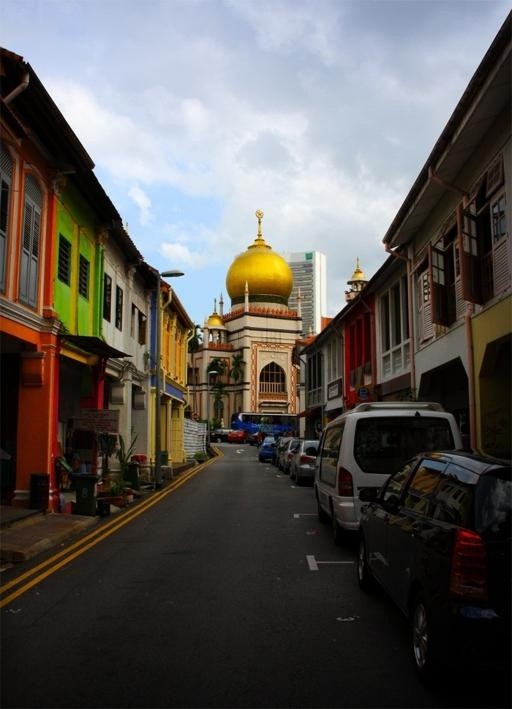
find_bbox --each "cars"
[288,439,321,486]
[270,436,302,475]
[258,435,276,463]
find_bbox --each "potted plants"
[194,451,207,465]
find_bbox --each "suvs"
[356,446,511,688]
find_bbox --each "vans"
[305,400,464,546]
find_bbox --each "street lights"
[207,371,219,443]
[154,270,184,488]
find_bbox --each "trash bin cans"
[70,476,100,516]
[29,473,49,510]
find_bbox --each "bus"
[230,411,300,438]
